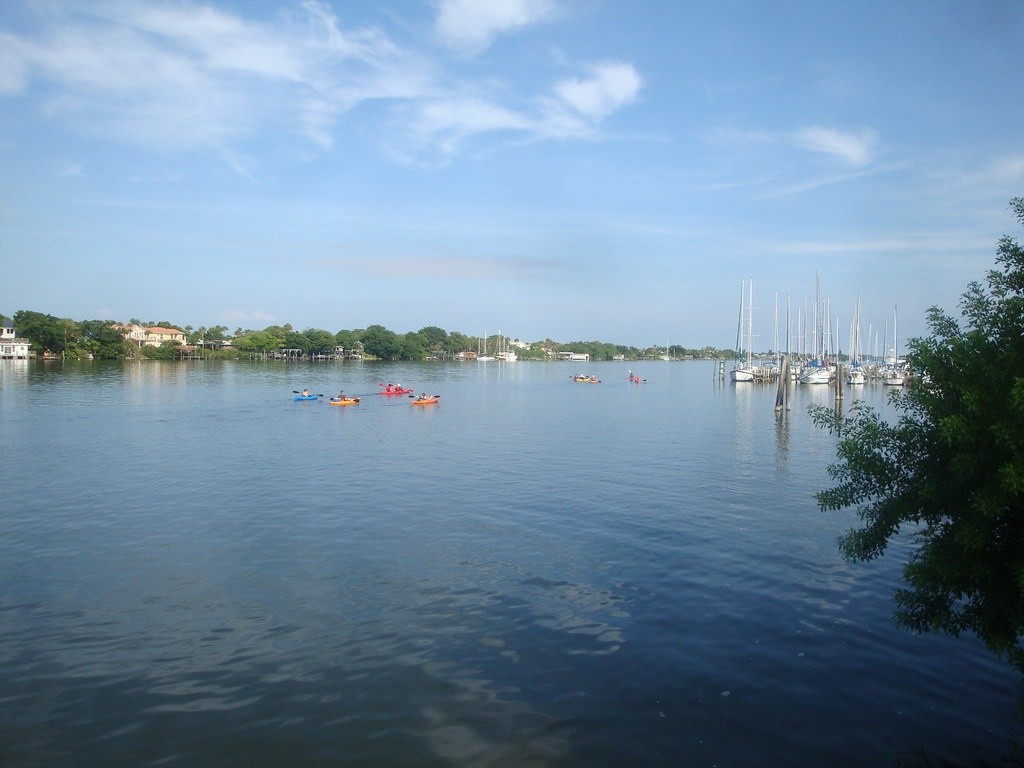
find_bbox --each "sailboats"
[474,327,517,363]
[728,275,914,386]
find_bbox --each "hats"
[389,384,393,386]
[397,384,400,386]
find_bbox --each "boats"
[575,377,599,383]
[380,388,414,395]
[412,396,440,404]
[296,395,317,400]
[329,399,360,406]
[629,375,640,384]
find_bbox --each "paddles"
[567,376,575,379]
[329,397,361,403]
[595,379,602,384]
[293,389,324,397]
[379,383,412,393]
[408,393,441,398]
[640,379,646,382]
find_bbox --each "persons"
[386,384,394,392]
[422,393,428,399]
[630,373,634,378]
[591,375,595,381]
[338,390,347,401]
[395,384,401,392]
[303,389,311,397]
[575,373,585,379]
